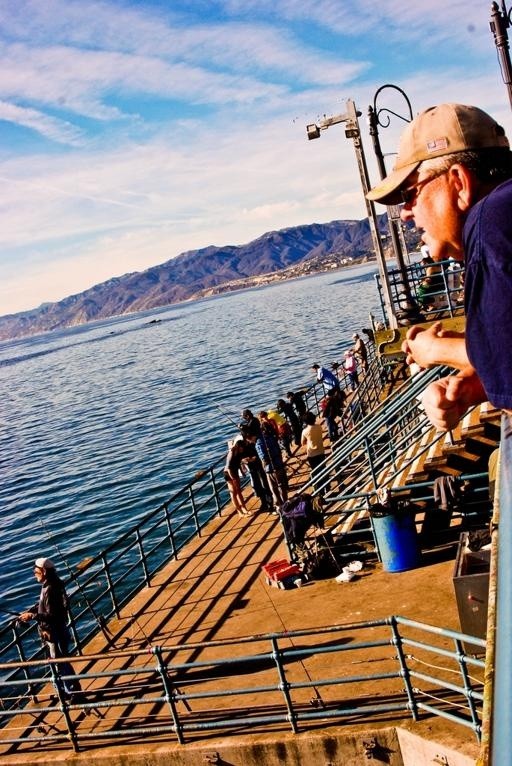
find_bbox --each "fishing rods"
[0,698,61,734]
[38,516,118,650]
[216,407,300,474]
[212,400,311,467]
[0,608,21,617]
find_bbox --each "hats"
[35,557,54,568]
[365,102,509,205]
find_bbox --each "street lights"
[302,94,398,327]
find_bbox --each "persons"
[365,103,512,431]
[344,321,383,393]
[397,257,461,324]
[17,557,88,706]
[223,363,348,519]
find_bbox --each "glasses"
[397,167,451,206]
[33,569,41,574]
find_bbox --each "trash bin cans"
[368,500,423,573]
